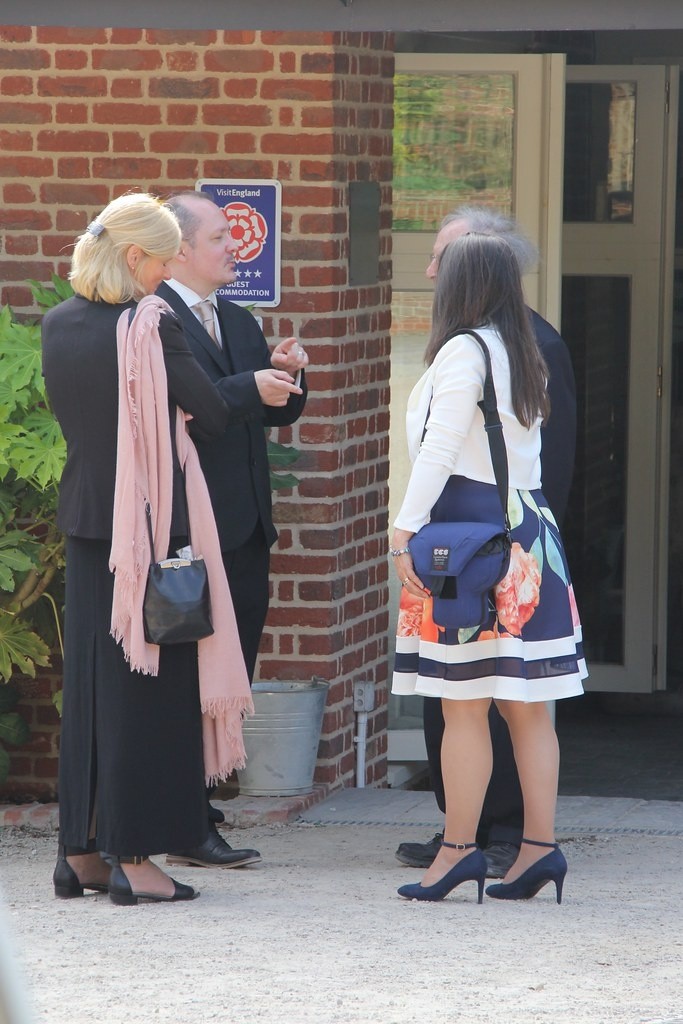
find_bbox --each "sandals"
[109,855,200,905]
[53,858,113,898]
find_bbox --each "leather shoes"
[166,826,263,869]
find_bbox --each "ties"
[192,301,221,353]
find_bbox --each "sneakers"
[395,832,444,868]
[482,843,520,878]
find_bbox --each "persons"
[389,230,588,905]
[394,209,577,878]
[155,194,310,870]
[40,192,256,905]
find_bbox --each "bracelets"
[390,546,411,556]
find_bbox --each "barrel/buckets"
[234,675,330,796]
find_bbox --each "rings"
[299,352,304,357]
[403,578,409,585]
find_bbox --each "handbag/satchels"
[408,522,510,627]
[144,555,214,645]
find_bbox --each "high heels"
[485,838,567,905]
[397,840,486,904]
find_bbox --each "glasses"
[429,253,441,263]
